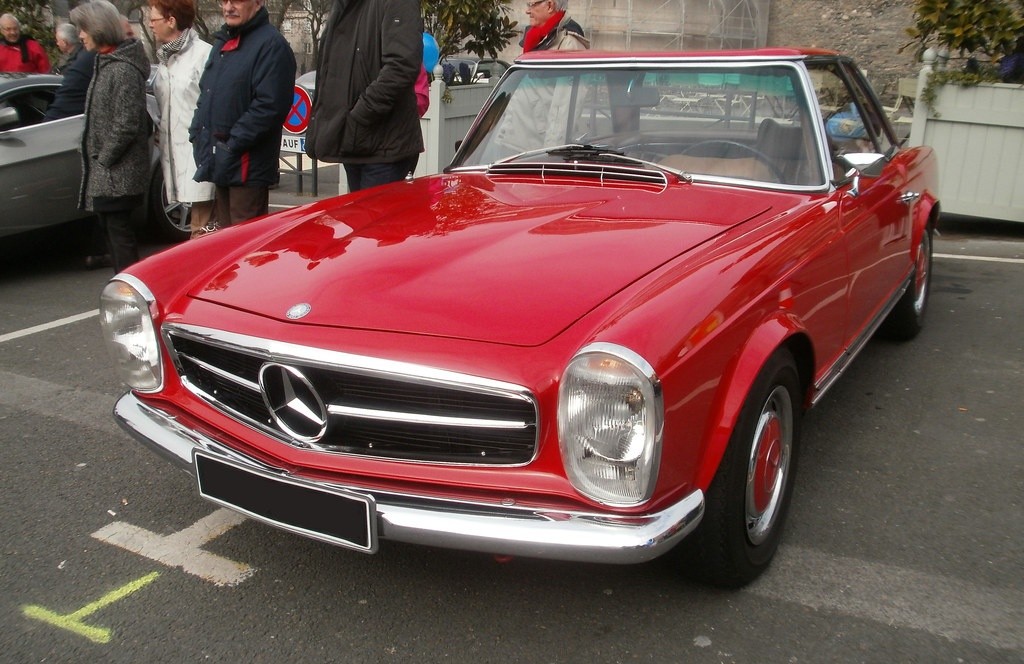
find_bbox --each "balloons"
[419,31,441,77]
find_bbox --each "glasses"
[151,16,169,25]
[55,39,66,43]
[1,28,19,31]
[528,0,547,7]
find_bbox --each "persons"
[189,0,296,227]
[519,0,593,58]
[144,1,216,239]
[1,0,152,275]
[305,1,431,195]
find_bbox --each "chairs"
[755,118,846,185]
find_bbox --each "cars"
[96,49,944,591]
[442,57,512,84]
[0,72,190,243]
